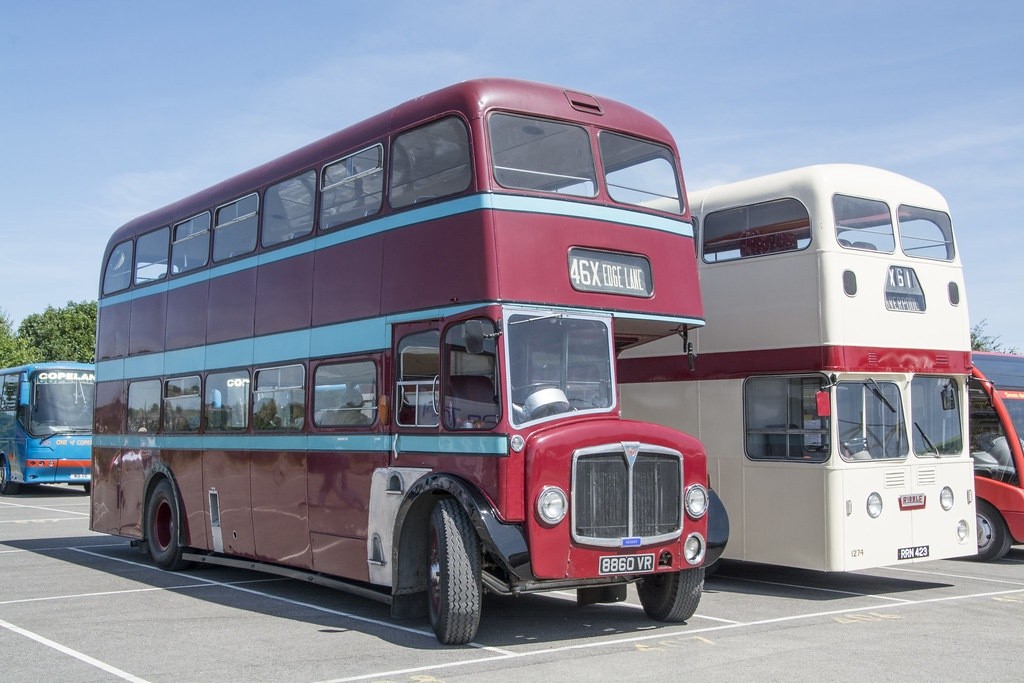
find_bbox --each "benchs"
[157,196,436,281]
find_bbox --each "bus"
[169,384,366,426]
[969,350,1024,563]
[362,164,977,572]
[90,76,729,647]
[0,361,97,495]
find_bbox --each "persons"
[253,398,304,431]
[324,390,371,425]
[317,453,365,514]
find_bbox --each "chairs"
[838,239,876,251]
[741,231,798,259]
